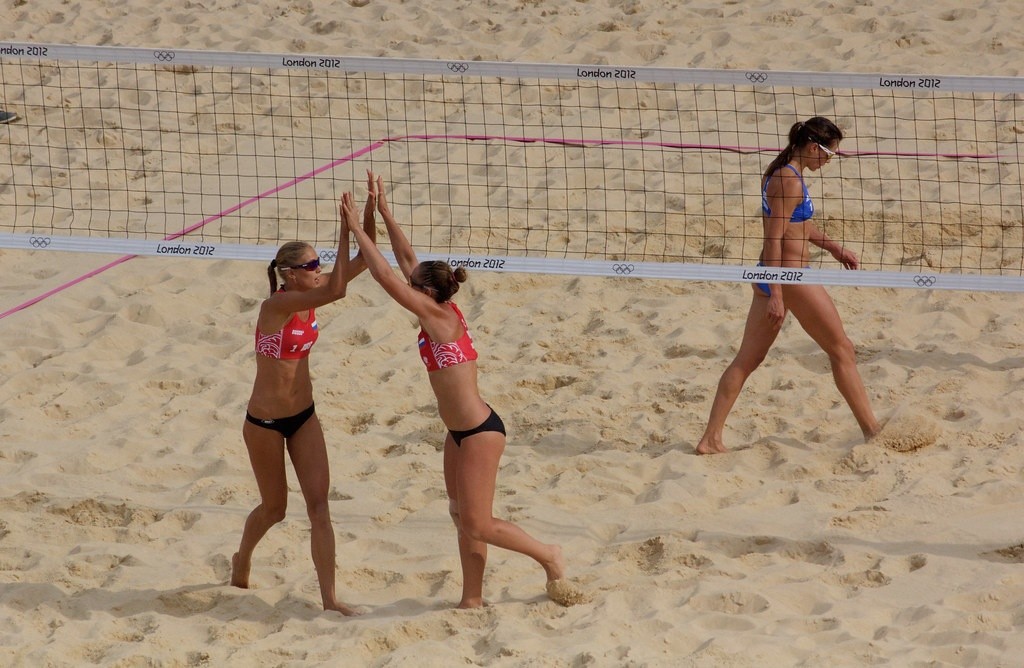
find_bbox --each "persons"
[231,168,378,616]
[696,117,880,456]
[343,178,569,609]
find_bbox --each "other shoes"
[0,109,17,124]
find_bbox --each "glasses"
[281,257,320,271]
[808,136,836,159]
[408,276,424,290]
